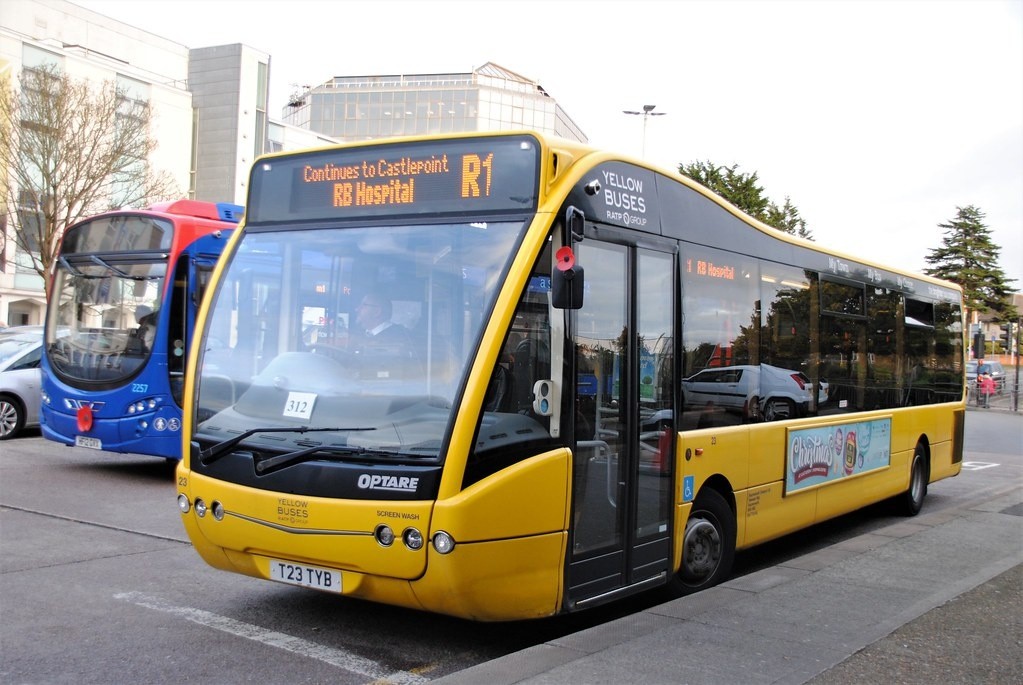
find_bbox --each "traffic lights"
[790,325,800,336]
[876,308,893,355]
[838,324,850,354]
[999,323,1011,350]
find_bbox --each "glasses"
[358,302,380,309]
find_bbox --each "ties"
[366,331,373,339]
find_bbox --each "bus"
[41,199,613,461]
[177,127,966,626]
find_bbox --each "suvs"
[964,360,1006,396]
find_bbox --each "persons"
[347,287,423,383]
[978,372,1000,408]
[134,305,157,352]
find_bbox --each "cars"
[682,366,831,421]
[0,326,125,440]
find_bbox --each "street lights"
[622,103,667,163]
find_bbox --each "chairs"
[510,340,552,418]
